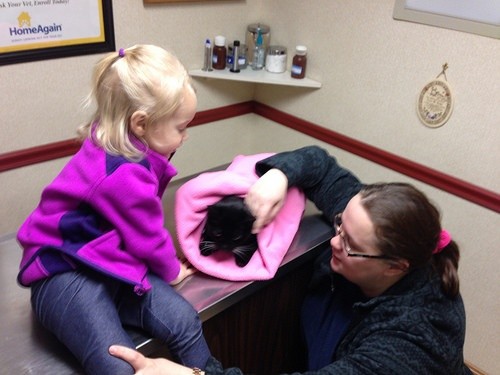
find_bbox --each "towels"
[173,152,307,282]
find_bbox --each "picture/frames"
[0,0,116,67]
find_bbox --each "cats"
[198,195,260,267]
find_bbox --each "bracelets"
[192,367,201,375]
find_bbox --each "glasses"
[334,213,402,261]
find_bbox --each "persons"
[16,44,243,375]
[108,145,474,375]
